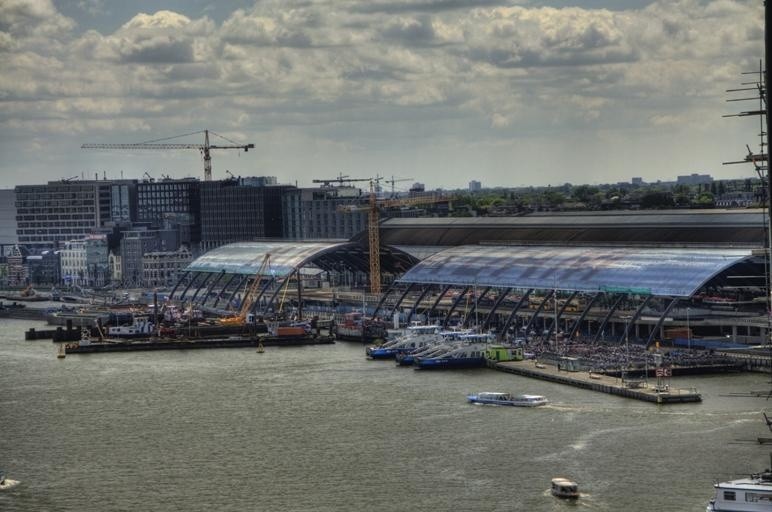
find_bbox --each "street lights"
[640,353,650,387]
[620,314,634,363]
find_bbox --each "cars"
[523,351,538,360]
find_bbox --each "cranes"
[78,126,257,181]
[311,169,372,185]
[333,179,460,296]
[373,172,384,183]
[384,174,415,191]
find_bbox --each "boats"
[394,331,463,366]
[0,290,338,354]
[465,391,549,408]
[549,476,581,501]
[332,312,388,345]
[413,333,490,371]
[706,468,772,512]
[365,325,443,360]
